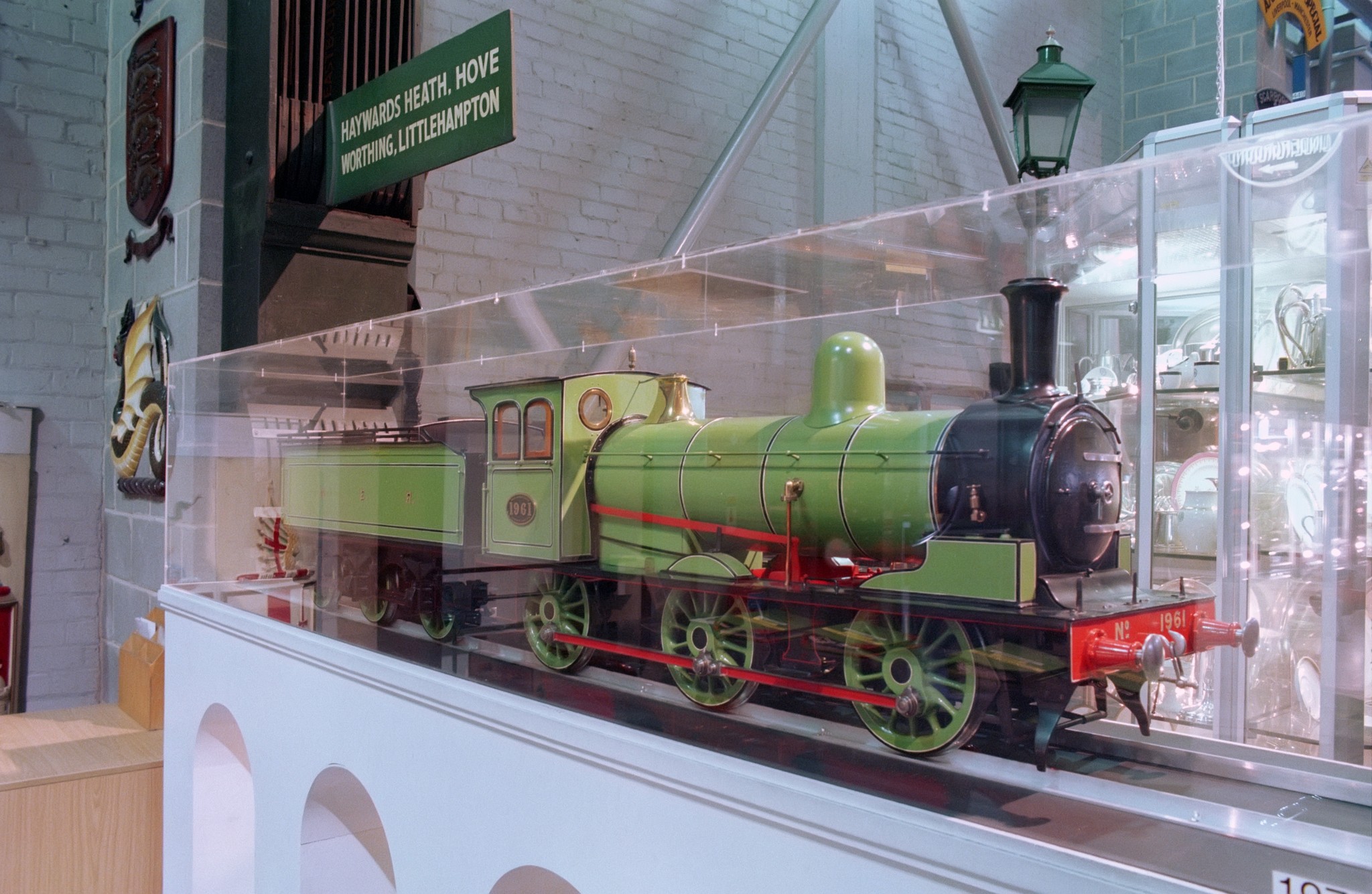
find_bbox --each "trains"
[247,279,1259,771]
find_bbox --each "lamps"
[1004,24,1097,250]
